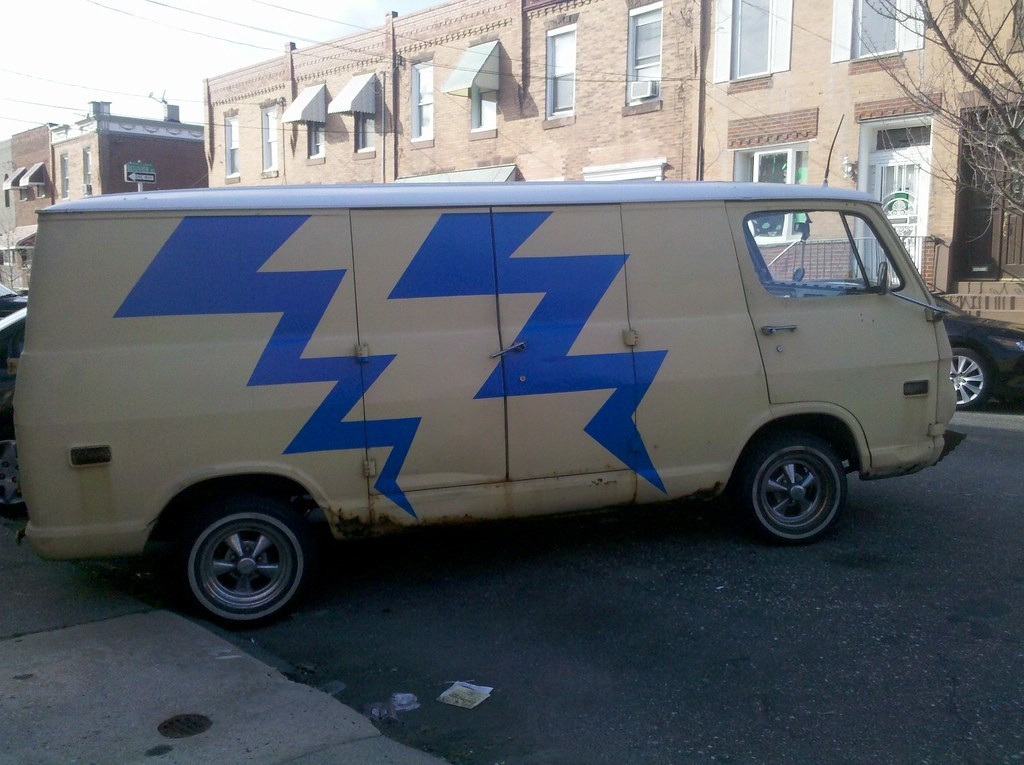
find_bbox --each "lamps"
[841,157,855,180]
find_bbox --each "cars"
[0,283,28,319]
[767,279,1024,409]
[0,306,32,507]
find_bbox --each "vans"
[10,182,956,631]
